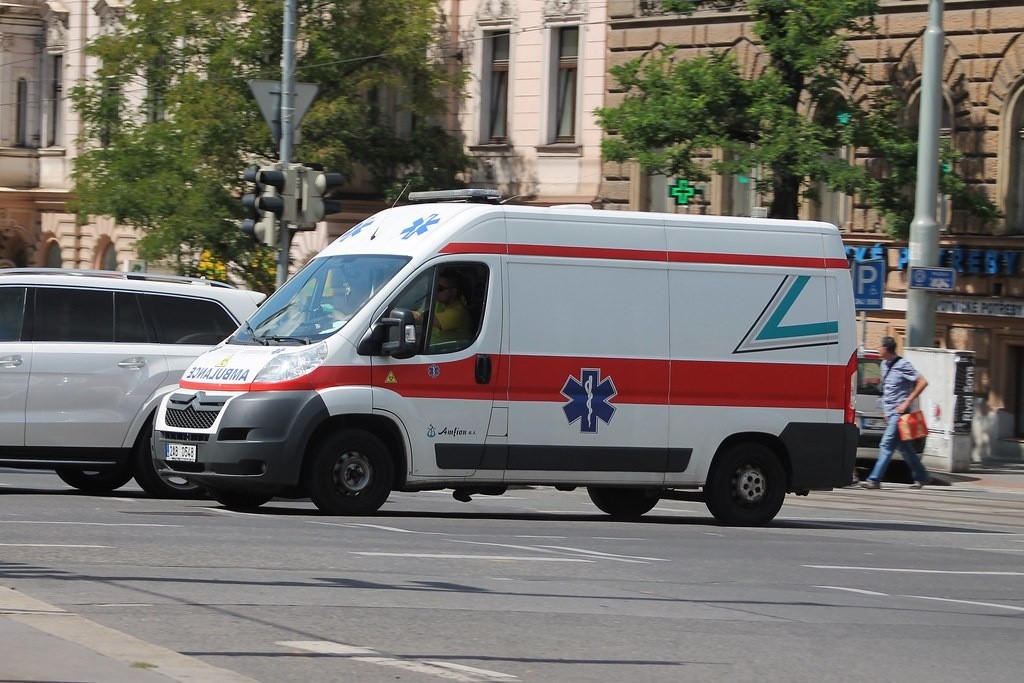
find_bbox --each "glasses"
[436,284,453,292]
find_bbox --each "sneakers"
[910,477,932,488]
[859,481,880,489]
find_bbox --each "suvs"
[0,267,268,499]
[854,350,927,462]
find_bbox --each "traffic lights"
[258,170,284,220]
[240,165,260,234]
[324,173,343,218]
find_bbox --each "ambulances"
[152,190,861,528]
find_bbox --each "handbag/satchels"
[898,409,928,441]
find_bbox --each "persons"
[970,372,1001,467]
[409,272,476,349]
[861,337,937,489]
